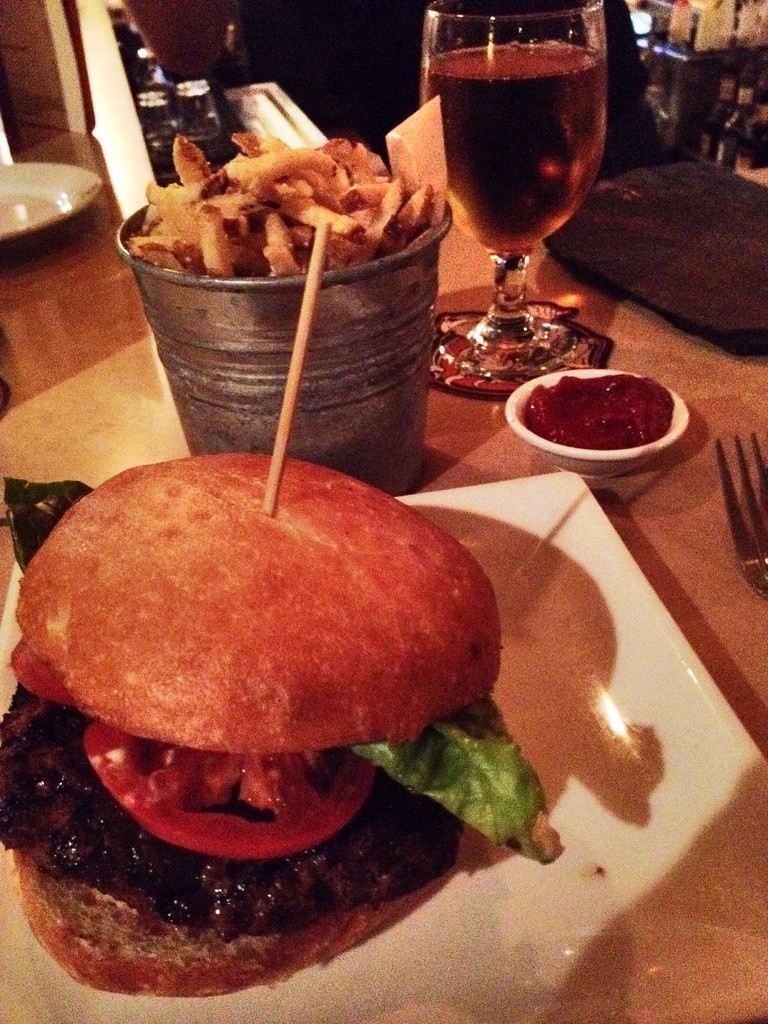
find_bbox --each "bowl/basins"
[503,369,690,480]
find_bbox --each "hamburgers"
[0,449,562,1001]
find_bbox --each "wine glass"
[426,0,607,398]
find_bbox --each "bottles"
[666,0,768,52]
[700,72,756,171]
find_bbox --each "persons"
[123,0,666,180]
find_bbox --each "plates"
[544,158,768,346]
[0,471,767,1024]
[0,163,103,241]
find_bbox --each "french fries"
[125,129,447,276]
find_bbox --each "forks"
[714,434,768,601]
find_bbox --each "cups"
[114,199,454,496]
[136,78,222,148]
